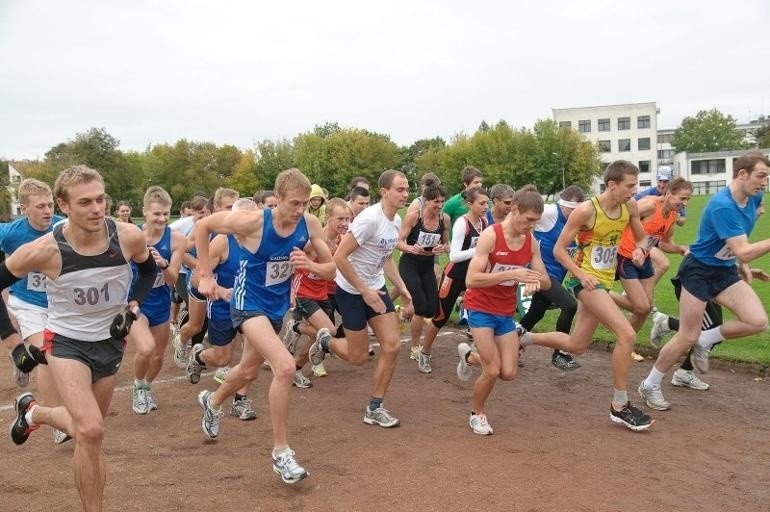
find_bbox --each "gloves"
[110,307,137,340]
[11,343,38,375]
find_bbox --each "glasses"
[499,198,513,205]
[291,201,312,208]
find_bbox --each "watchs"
[125,304,141,321]
[162,258,169,270]
[640,247,648,258]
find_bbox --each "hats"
[657,165,673,183]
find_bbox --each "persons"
[0,164,158,512]
[168,195,212,330]
[321,188,329,201]
[396,184,450,362]
[634,164,687,314]
[349,176,370,191]
[456,184,552,435]
[115,200,136,225]
[0,179,73,445]
[254,191,278,214]
[513,160,657,431]
[128,185,187,415]
[348,187,368,217]
[649,192,770,391]
[609,178,692,361]
[389,174,443,323]
[194,168,337,485]
[282,196,350,389]
[441,166,485,259]
[637,152,770,411]
[172,187,241,383]
[309,170,414,428]
[517,185,586,371]
[185,199,260,421]
[311,203,357,378]
[414,187,489,374]
[179,200,195,218]
[484,183,516,228]
[304,183,329,228]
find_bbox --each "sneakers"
[171,335,190,370]
[410,346,422,360]
[230,396,258,420]
[671,371,710,392]
[369,346,375,357]
[14,365,31,388]
[394,305,404,322]
[610,401,656,432]
[363,404,401,428]
[169,320,179,334]
[457,342,474,383]
[213,366,231,385]
[52,430,71,444]
[515,320,526,359]
[368,327,374,337]
[457,296,463,307]
[186,343,208,384]
[133,380,149,417]
[311,361,328,377]
[10,393,36,446]
[631,351,645,363]
[637,378,672,412]
[469,412,493,436]
[309,328,330,365]
[263,360,271,370]
[198,389,221,438]
[147,388,159,413]
[283,319,300,355]
[272,446,308,484]
[691,342,712,376]
[649,309,669,351]
[293,368,314,390]
[552,350,580,371]
[417,347,432,375]
